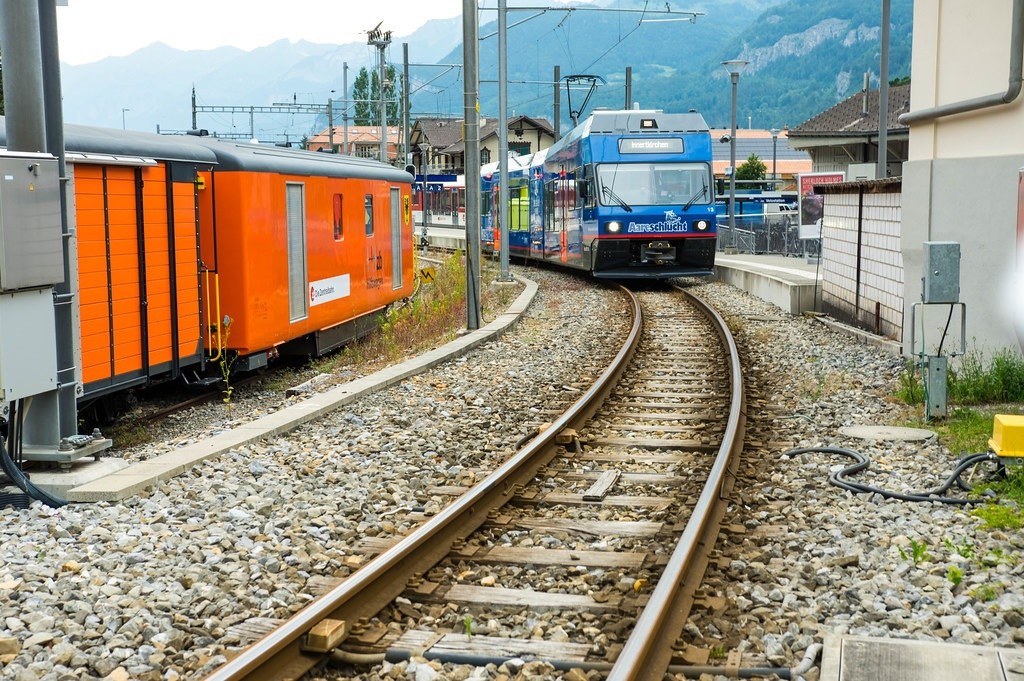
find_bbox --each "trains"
[416,103,725,284]
[1,115,417,427]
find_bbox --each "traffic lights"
[405,164,416,180]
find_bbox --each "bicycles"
[735,221,822,258]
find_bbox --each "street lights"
[770,128,780,191]
[720,60,751,247]
[418,142,431,253]
